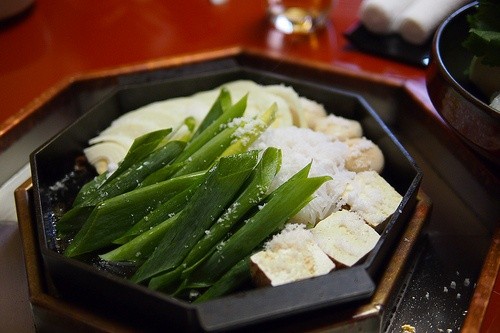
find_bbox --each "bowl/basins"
[425,0,499,155]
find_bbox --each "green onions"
[54,87,333,303]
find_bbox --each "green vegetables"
[457,0,499,91]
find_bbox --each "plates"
[341,18,433,68]
[0,45,499,333]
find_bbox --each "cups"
[265,0,336,37]
